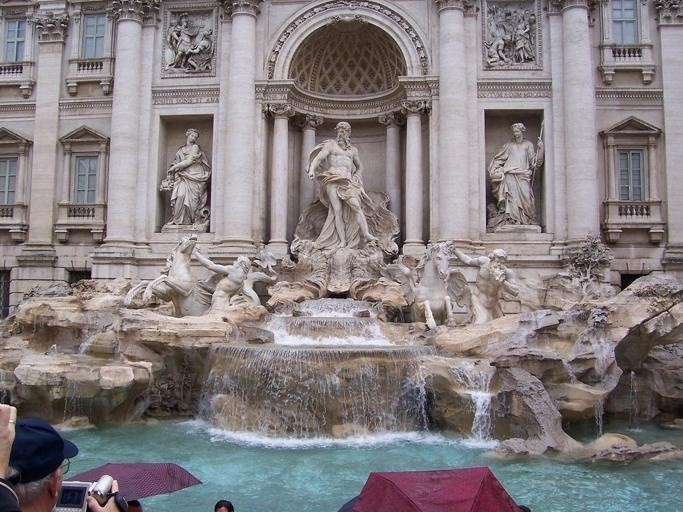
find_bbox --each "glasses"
[48,458,70,480]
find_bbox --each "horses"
[410,242,457,331]
[124,235,212,317]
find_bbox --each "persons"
[158,127,212,229]
[305,120,378,249]
[212,497,236,511]
[485,121,545,228]
[484,9,539,67]
[0,393,147,510]
[191,245,252,314]
[162,11,215,73]
[450,244,521,326]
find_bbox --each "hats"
[8,419,78,486]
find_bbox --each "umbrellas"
[62,459,201,506]
[333,465,525,512]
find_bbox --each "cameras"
[54,474,113,512]
